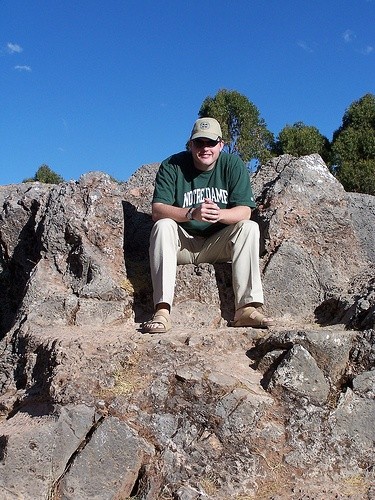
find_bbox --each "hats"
[191,117,222,141]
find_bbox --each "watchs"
[186,208,197,221]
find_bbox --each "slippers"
[141,310,173,333]
[229,306,275,327]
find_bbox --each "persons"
[144,118,276,332]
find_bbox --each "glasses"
[191,139,220,147]
